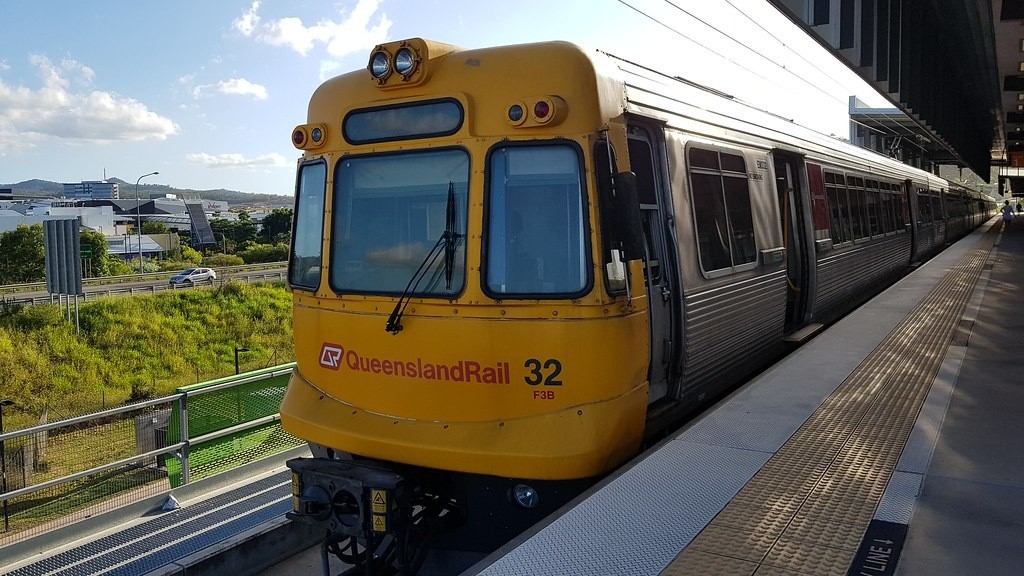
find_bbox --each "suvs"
[169,268,216,288]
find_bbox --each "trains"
[279,37,999,576]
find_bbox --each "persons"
[1016,203,1022,212]
[1001,200,1014,232]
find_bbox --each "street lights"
[135,171,160,275]
